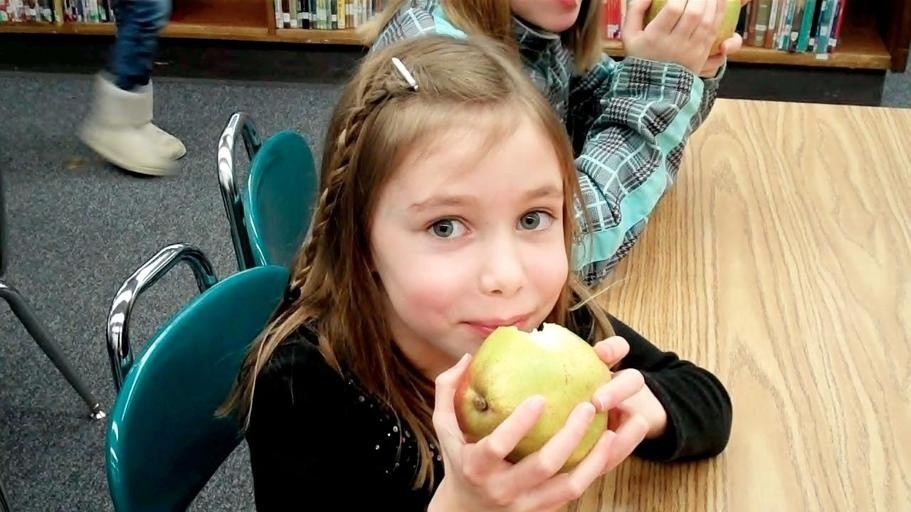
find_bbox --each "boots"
[72,69,193,180]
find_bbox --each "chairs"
[215,111,321,271]
[3,180,105,421]
[108,241,293,510]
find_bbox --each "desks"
[558,98,911,512]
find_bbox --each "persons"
[211,34,735,512]
[357,1,746,296]
[70,1,188,177]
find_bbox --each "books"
[601,0,846,52]
[276,0,375,30]
[1,1,119,30]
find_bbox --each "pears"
[460,324,613,475]
[643,0,741,55]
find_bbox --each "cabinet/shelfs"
[0,1,889,109]
